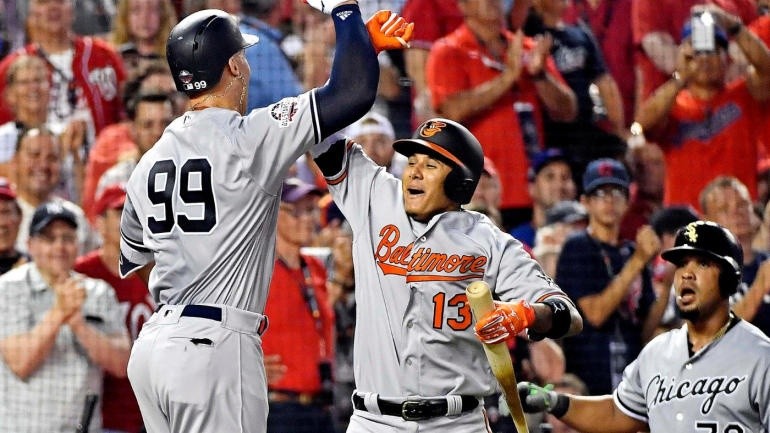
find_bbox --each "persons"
[307,11,584,433]
[0,0,770,433]
[118,0,379,433]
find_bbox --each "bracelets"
[530,67,546,81]
[728,21,742,36]
[672,70,686,88]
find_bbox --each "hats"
[582,158,630,193]
[347,114,394,140]
[653,208,698,236]
[92,159,136,215]
[284,176,326,204]
[0,178,16,200]
[30,204,78,235]
[482,157,497,178]
[681,19,729,47]
[531,148,572,174]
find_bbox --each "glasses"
[281,206,321,219]
[593,188,623,197]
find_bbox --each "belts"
[353,394,496,420]
[155,304,222,322]
[268,390,313,406]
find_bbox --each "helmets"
[392,118,483,204]
[166,9,260,92]
[661,220,744,297]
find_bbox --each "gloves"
[474,299,537,345]
[499,382,570,419]
[307,0,358,14]
[365,9,415,56]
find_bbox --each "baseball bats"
[465,281,529,433]
